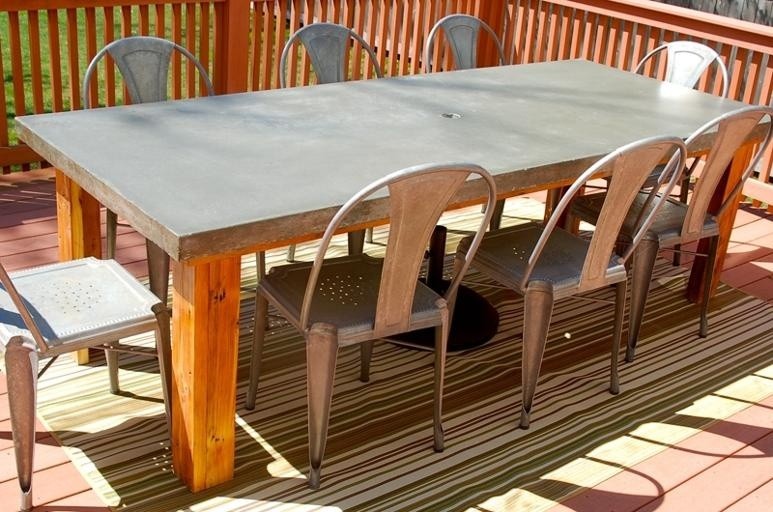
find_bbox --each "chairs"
[0,255,175,510]
[244,160,498,490]
[447,134,687,429]
[602,42,729,266]
[424,13,506,231]
[82,37,270,328]
[278,23,383,263]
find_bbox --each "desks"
[14,58,773,493]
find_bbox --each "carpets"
[0,196,771,509]
